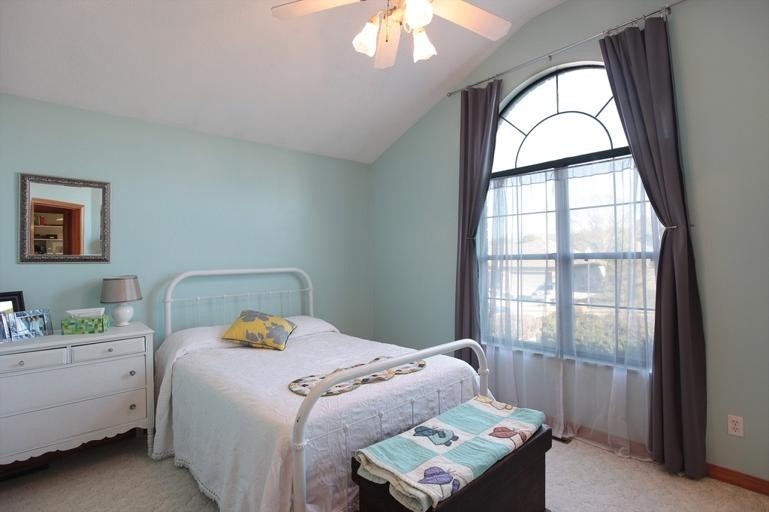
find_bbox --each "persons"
[20,316,44,333]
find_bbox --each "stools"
[351,395,552,512]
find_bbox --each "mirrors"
[18,172,111,263]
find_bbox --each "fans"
[268,0,513,72]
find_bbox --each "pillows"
[222,307,298,351]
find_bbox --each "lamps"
[351,0,438,64]
[99,274,144,328]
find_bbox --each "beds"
[156,267,489,511]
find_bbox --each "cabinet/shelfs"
[0,320,156,466]
[34,212,64,256]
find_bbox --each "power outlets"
[726,414,744,438]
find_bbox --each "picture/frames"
[0,290,56,343]
